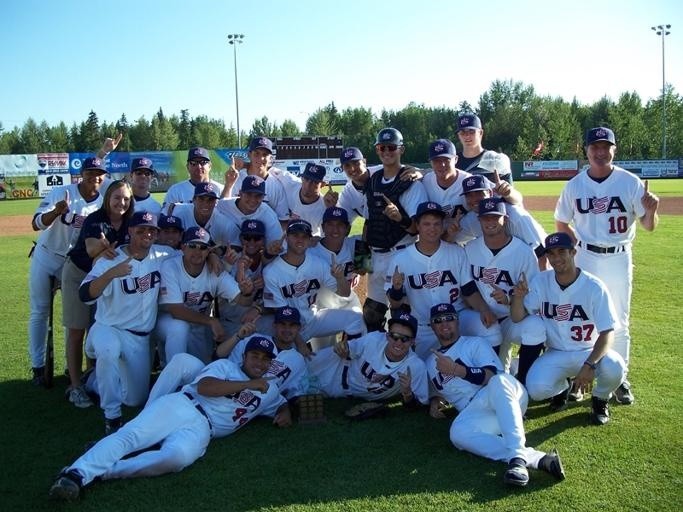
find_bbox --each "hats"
[480,198,507,217]
[158,216,185,232]
[275,306,302,326]
[388,312,418,333]
[287,220,312,238]
[339,147,364,163]
[375,128,392,145]
[459,176,492,195]
[301,163,327,183]
[546,232,575,250]
[250,136,274,154]
[431,304,462,316]
[181,227,213,245]
[187,148,212,163]
[456,113,483,132]
[242,176,267,195]
[130,157,155,174]
[195,183,223,200]
[584,127,615,145]
[81,158,110,176]
[413,201,445,217]
[246,337,278,358]
[240,220,265,236]
[323,205,348,223]
[129,211,161,230]
[432,140,456,159]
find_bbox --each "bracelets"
[585,360,598,370]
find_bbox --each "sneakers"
[615,385,635,405]
[69,389,93,407]
[569,387,585,400]
[104,418,121,434]
[505,462,529,485]
[31,367,47,382]
[591,393,613,428]
[61,472,82,502]
[541,447,566,478]
[50,482,66,500]
[551,380,573,411]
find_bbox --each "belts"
[578,241,633,254]
[342,354,354,392]
[181,391,215,441]
[370,245,411,255]
[119,325,156,337]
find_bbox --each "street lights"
[650,24,671,160]
[227,32,245,148]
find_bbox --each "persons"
[144,305,307,427]
[262,221,367,362]
[90,135,162,244]
[425,303,567,486]
[76,212,226,434]
[324,150,425,238]
[48,335,292,495]
[154,228,252,367]
[457,115,511,186]
[511,233,627,427]
[464,202,546,385]
[357,127,433,333]
[553,126,659,403]
[188,219,296,363]
[25,155,110,385]
[442,177,546,274]
[423,140,522,222]
[168,183,243,257]
[63,182,135,407]
[302,313,428,408]
[163,147,222,212]
[215,138,293,233]
[232,154,324,237]
[211,176,287,256]
